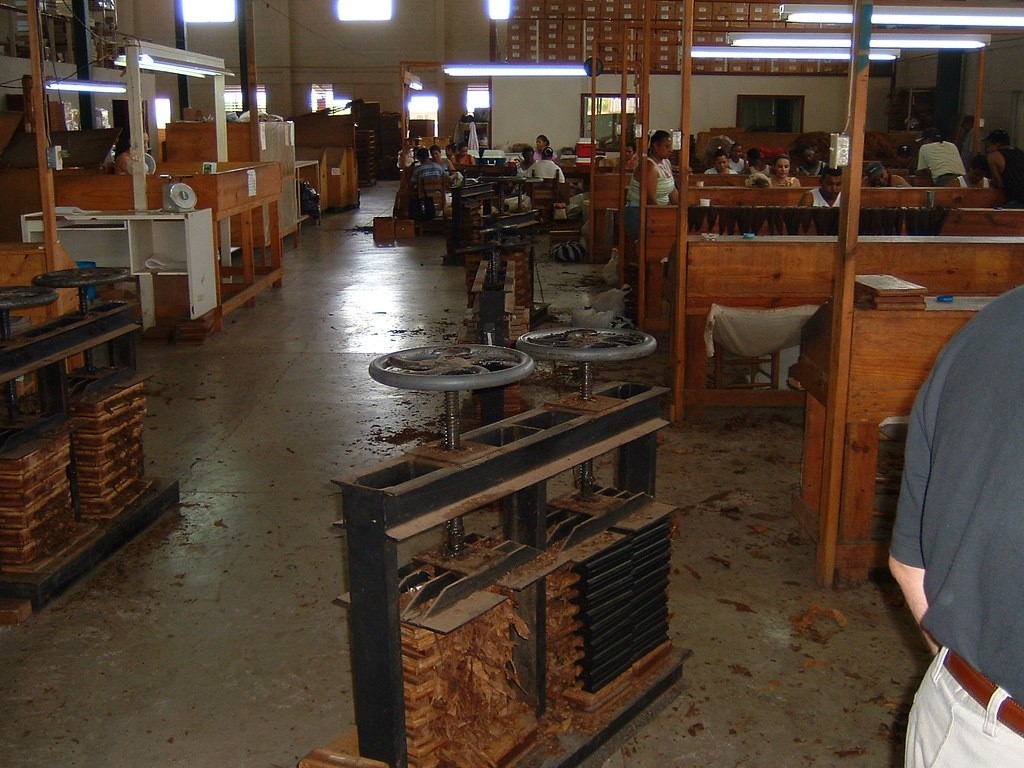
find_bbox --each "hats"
[864,161,884,182]
[745,173,771,188]
[980,128,1009,143]
[897,144,911,158]
[960,115,974,127]
[915,127,940,142]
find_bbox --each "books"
[854,273,927,310]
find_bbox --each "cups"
[696,181,704,186]
[700,199,710,206]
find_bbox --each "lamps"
[114,55,235,78]
[726,32,991,48]
[690,46,901,61]
[780,4,1024,26]
[45,80,127,93]
[440,60,589,76]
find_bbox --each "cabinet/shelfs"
[21,208,217,331]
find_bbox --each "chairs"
[417,170,447,233]
[527,169,560,226]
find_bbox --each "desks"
[558,164,1024,586]
[0,121,359,397]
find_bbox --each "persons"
[861,161,912,187]
[429,145,457,185]
[409,148,443,232]
[981,129,1024,209]
[527,147,566,228]
[912,116,992,188]
[727,143,746,174]
[533,135,558,161]
[798,165,842,207]
[445,143,459,169]
[768,153,802,187]
[704,149,738,174]
[741,148,775,174]
[507,146,536,197]
[114,124,156,175]
[888,283,1024,768]
[794,144,829,176]
[622,130,678,278]
[456,142,476,165]
[612,140,638,173]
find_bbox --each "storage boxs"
[428,149,507,167]
[355,113,434,187]
[395,219,414,238]
[374,217,395,240]
[550,230,579,246]
[560,138,600,173]
[508,0,854,72]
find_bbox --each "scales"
[159,174,198,213]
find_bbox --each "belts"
[943,649,1024,740]
